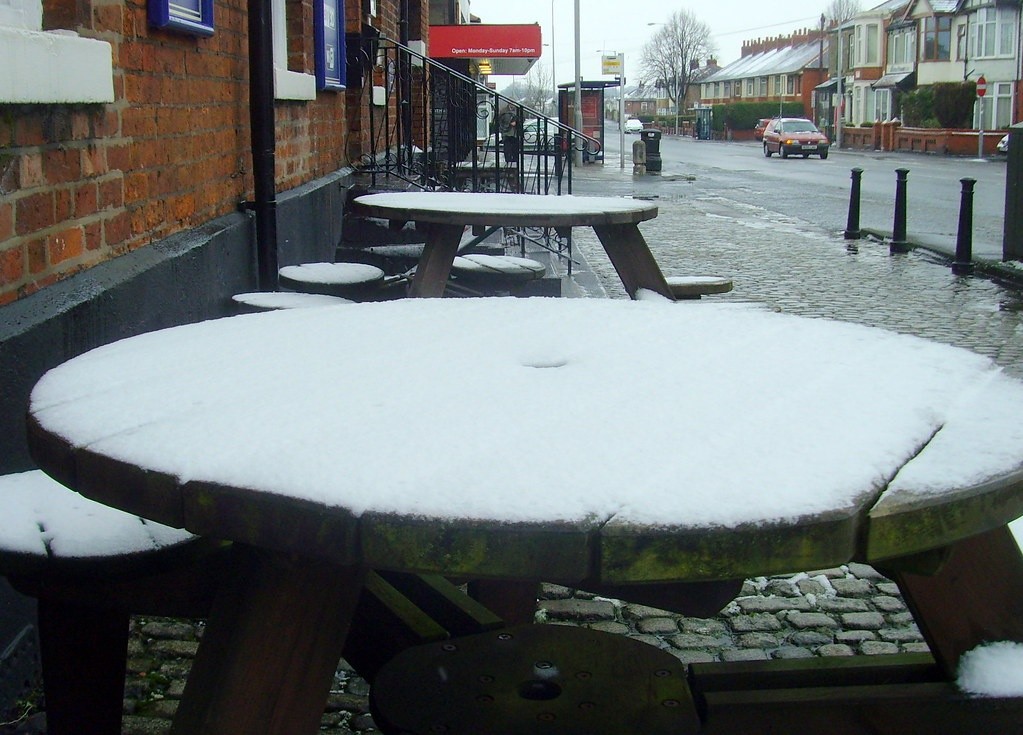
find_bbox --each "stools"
[233,292,355,315]
[666,277,733,300]
[0,471,212,735]
[277,262,385,294]
[451,255,545,296]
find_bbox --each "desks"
[355,192,675,300]
[28,293,1022,735]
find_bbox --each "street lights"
[647,22,679,137]
[596,50,617,98]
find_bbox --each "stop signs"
[977,77,987,97]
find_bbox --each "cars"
[763,118,829,160]
[754,119,771,141]
[484,117,559,151]
[625,119,643,133]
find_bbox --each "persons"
[500,96,526,162]
[695,117,702,140]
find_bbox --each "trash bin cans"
[639,129,662,171]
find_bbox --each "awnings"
[814,78,845,89]
[872,72,913,88]
